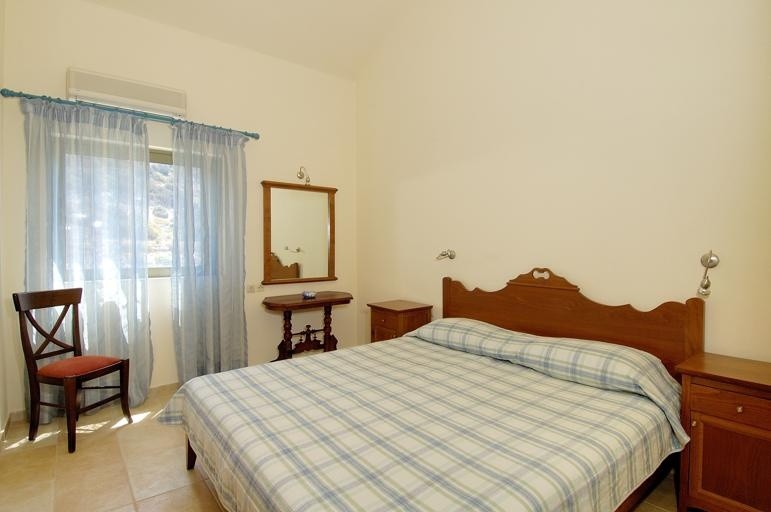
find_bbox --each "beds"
[152,265,707,512]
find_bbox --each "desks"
[262,290,354,363]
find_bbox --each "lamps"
[296,165,312,187]
[434,247,457,264]
[697,248,722,299]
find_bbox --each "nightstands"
[674,348,771,512]
[366,298,434,344]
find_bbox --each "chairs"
[12,288,136,455]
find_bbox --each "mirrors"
[260,178,340,284]
